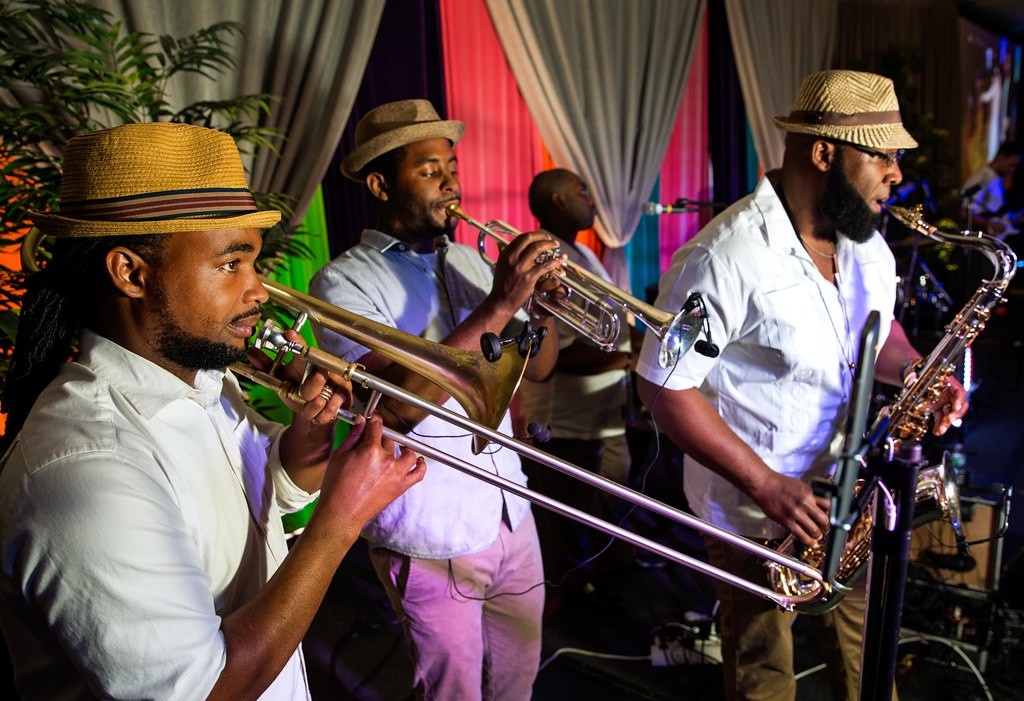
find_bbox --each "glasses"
[829,140,905,166]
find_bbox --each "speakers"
[904,481,1011,604]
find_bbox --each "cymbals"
[889,232,940,248]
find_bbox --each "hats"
[25,122,282,236]
[774,70,920,150]
[341,99,466,182]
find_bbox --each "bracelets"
[899,356,924,381]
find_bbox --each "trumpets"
[447,198,709,370]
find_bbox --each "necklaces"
[799,232,839,274]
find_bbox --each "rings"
[317,387,333,400]
[942,404,951,409]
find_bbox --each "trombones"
[223,269,830,614]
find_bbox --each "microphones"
[689,291,719,357]
[641,201,690,214]
[515,421,552,443]
[956,533,978,572]
[961,175,990,200]
[480,331,520,362]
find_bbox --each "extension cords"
[666,632,724,665]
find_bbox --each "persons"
[0,121,427,701]
[512,167,632,594]
[634,70,970,701]
[307,98,569,701]
[961,140,1024,289]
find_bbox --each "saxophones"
[765,196,1017,605]
